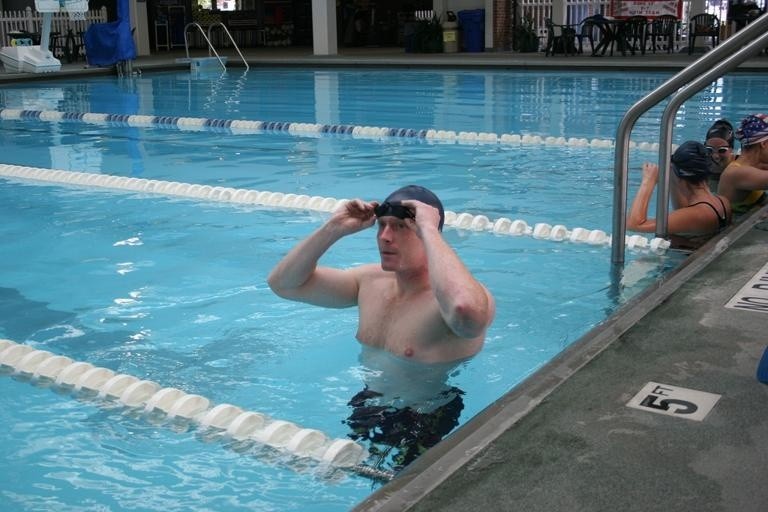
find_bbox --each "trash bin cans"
[442,22,458,52]
[458,9,485,52]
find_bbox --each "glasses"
[706,147,729,153]
[374,203,415,221]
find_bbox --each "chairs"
[14,23,137,65]
[507,11,722,57]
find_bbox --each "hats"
[735,113,768,145]
[673,141,713,177]
[382,185,444,232]
[706,121,734,149]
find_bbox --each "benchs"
[197,7,267,51]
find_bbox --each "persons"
[626,140,733,269]
[703,119,739,194]
[267,185,495,474]
[717,112,768,226]
